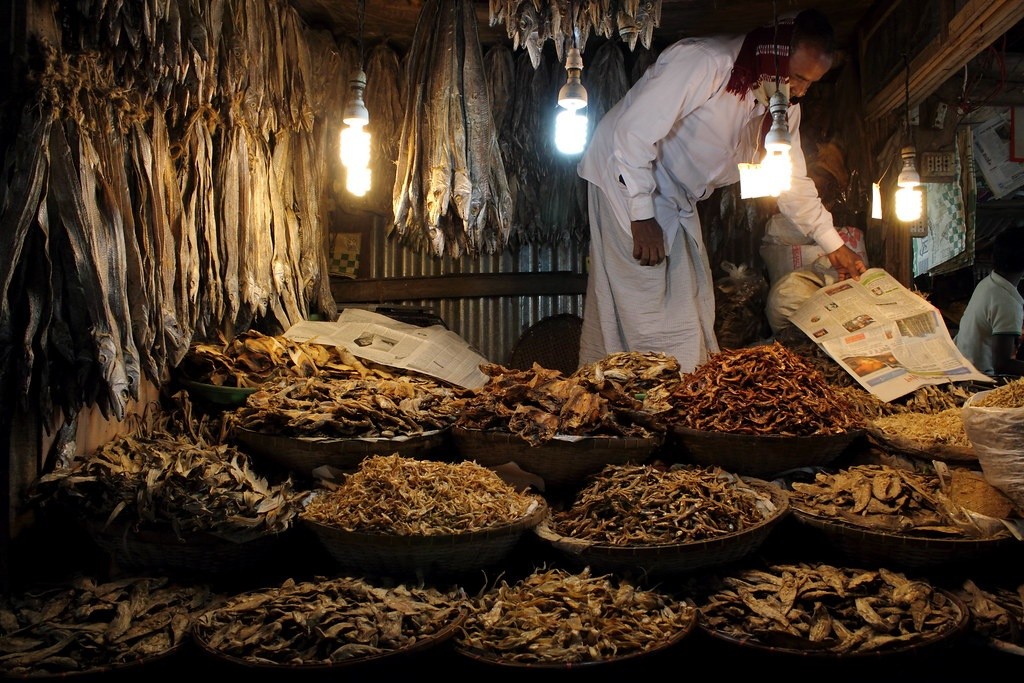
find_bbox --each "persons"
[953,228,1024,375]
[578,0,868,374]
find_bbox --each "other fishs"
[0,0,758,438]
[0,327,1024,683]
